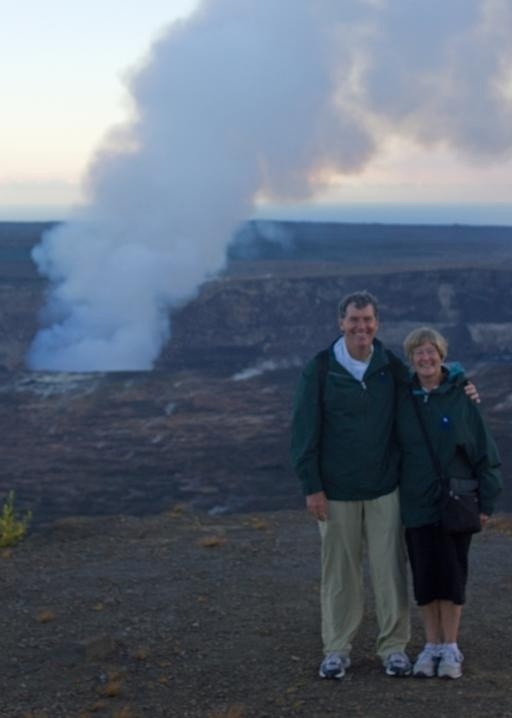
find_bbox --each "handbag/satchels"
[437,477,483,535]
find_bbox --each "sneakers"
[413,646,462,679]
[382,650,411,676]
[318,652,351,678]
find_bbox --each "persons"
[288,290,482,681]
[390,328,507,684]
